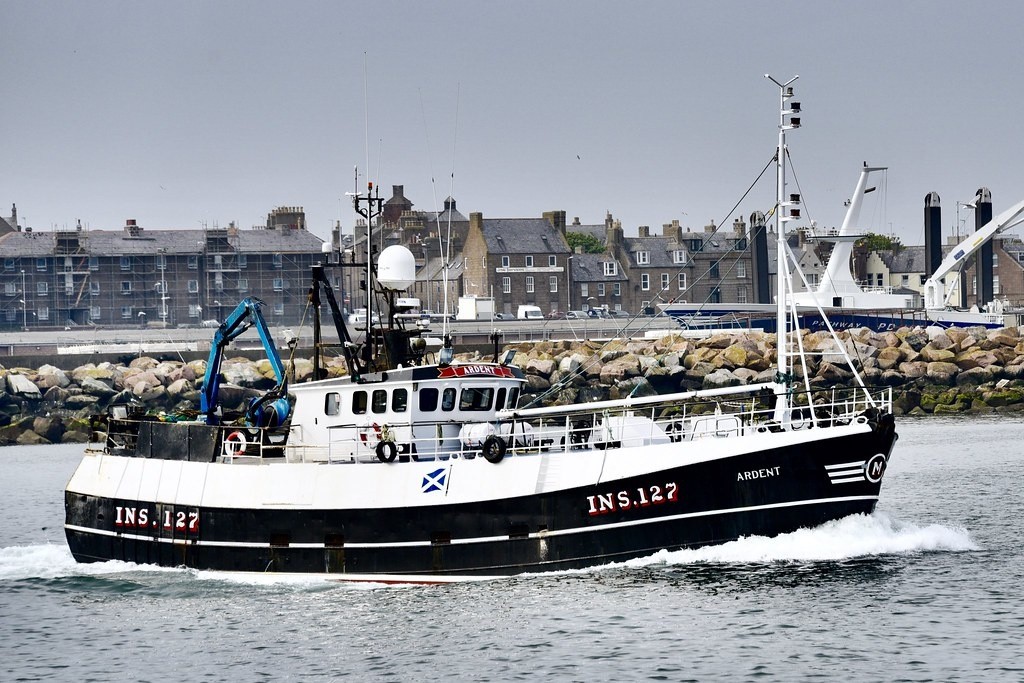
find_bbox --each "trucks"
[455,296,495,322]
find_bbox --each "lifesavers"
[359,421,382,448]
[666,424,686,443]
[225,431,246,458]
[376,440,397,462]
[482,436,506,463]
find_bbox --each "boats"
[61,75,900,574]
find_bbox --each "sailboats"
[654,159,1020,332]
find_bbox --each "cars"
[493,312,518,321]
[560,311,590,320]
[587,306,629,319]
[348,308,381,325]
[543,311,569,321]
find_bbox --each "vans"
[517,304,544,320]
